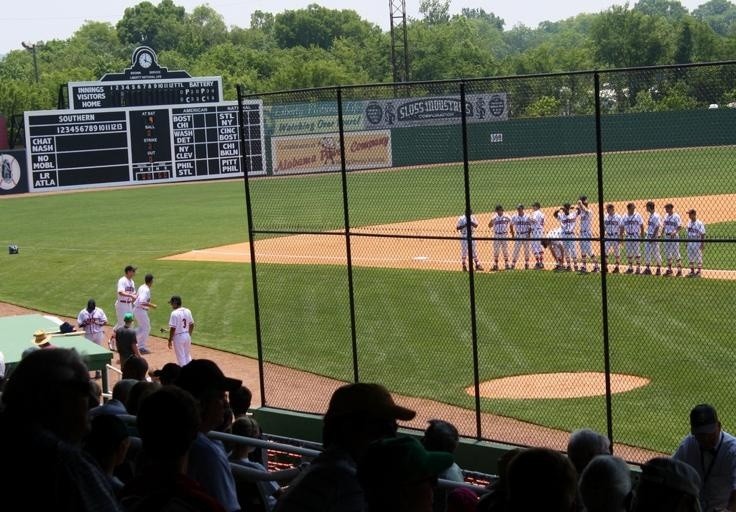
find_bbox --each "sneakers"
[476,265,484,271]
[612,266,700,277]
[492,263,600,273]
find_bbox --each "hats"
[541,238,552,248]
[168,296,182,305]
[328,382,417,420]
[125,265,137,273]
[374,437,455,479]
[606,202,696,215]
[495,196,587,210]
[690,404,718,434]
[124,313,133,321]
[175,358,242,396]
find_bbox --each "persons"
[487,205,511,271]
[456,206,484,271]
[540,228,566,272]
[273,383,465,512]
[576,195,601,273]
[509,204,532,270]
[168,295,195,367]
[477,404,736,512]
[553,203,581,271]
[133,274,157,355]
[683,208,708,277]
[33,329,56,349]
[621,203,645,275]
[115,313,141,380]
[603,203,623,274]
[529,202,547,269]
[78,299,108,379]
[105,265,136,351]
[642,201,665,276]
[1,348,286,512]
[658,203,684,277]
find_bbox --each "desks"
[0,314,114,403]
